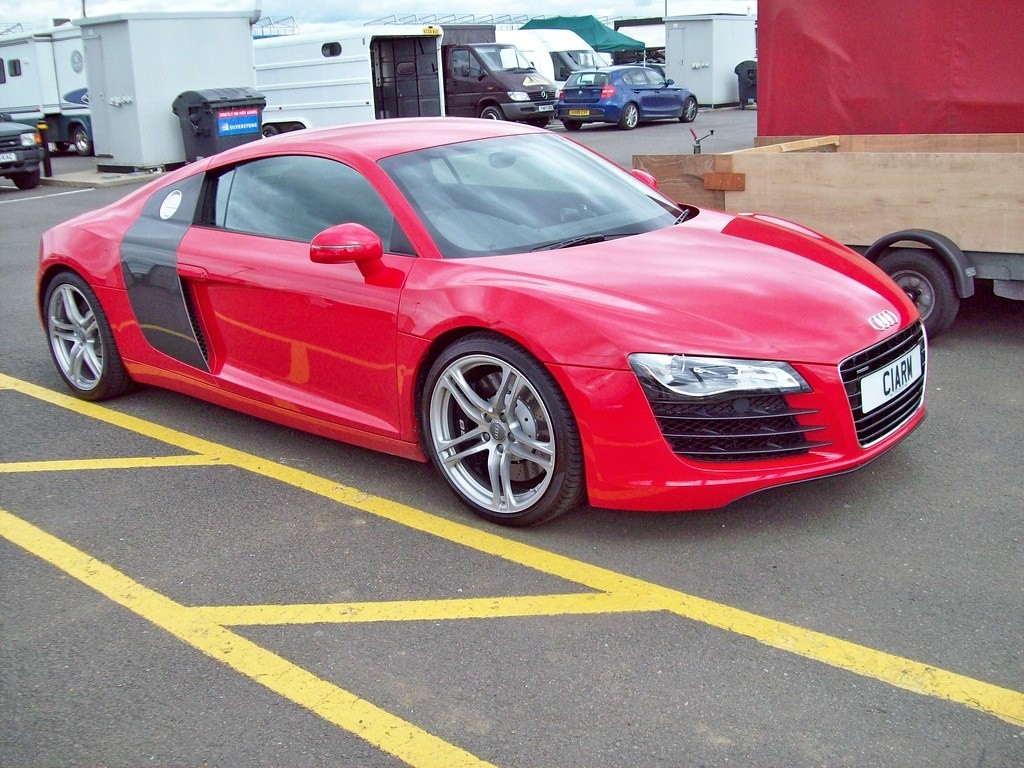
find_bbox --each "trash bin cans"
[170,85,267,165]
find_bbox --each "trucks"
[501,29,609,100]
[441,31,559,122]
[252,13,447,133]
[0,25,95,156]
[633,136,1024,338]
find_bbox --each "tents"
[517,14,646,67]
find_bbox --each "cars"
[34,116,929,528]
[0,110,47,190]
[553,65,698,131]
